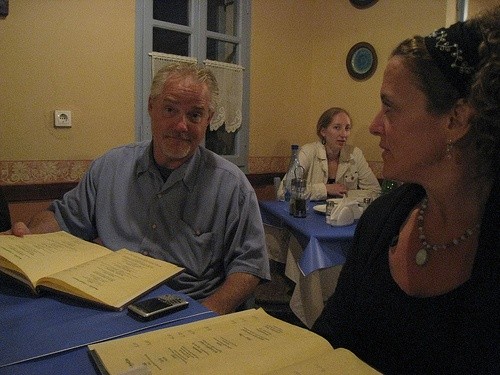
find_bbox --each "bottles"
[289,178,297,216]
[354,196,372,220]
[284,144,304,202]
[326,200,335,224]
[293,178,307,218]
[380,177,397,196]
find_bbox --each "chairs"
[241,265,300,326]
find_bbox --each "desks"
[259,198,361,276]
[0,273,219,374]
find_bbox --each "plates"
[346,41,378,79]
[349,0,378,9]
[313,204,339,215]
[326,198,344,204]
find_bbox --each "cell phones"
[127,294,189,321]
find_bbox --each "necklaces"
[415,208,483,267]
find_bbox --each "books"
[0,231,185,311]
[86,307,384,375]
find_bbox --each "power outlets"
[55,109,71,127]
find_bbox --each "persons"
[313,10,500,375]
[0,59,271,314]
[278,108,382,204]
